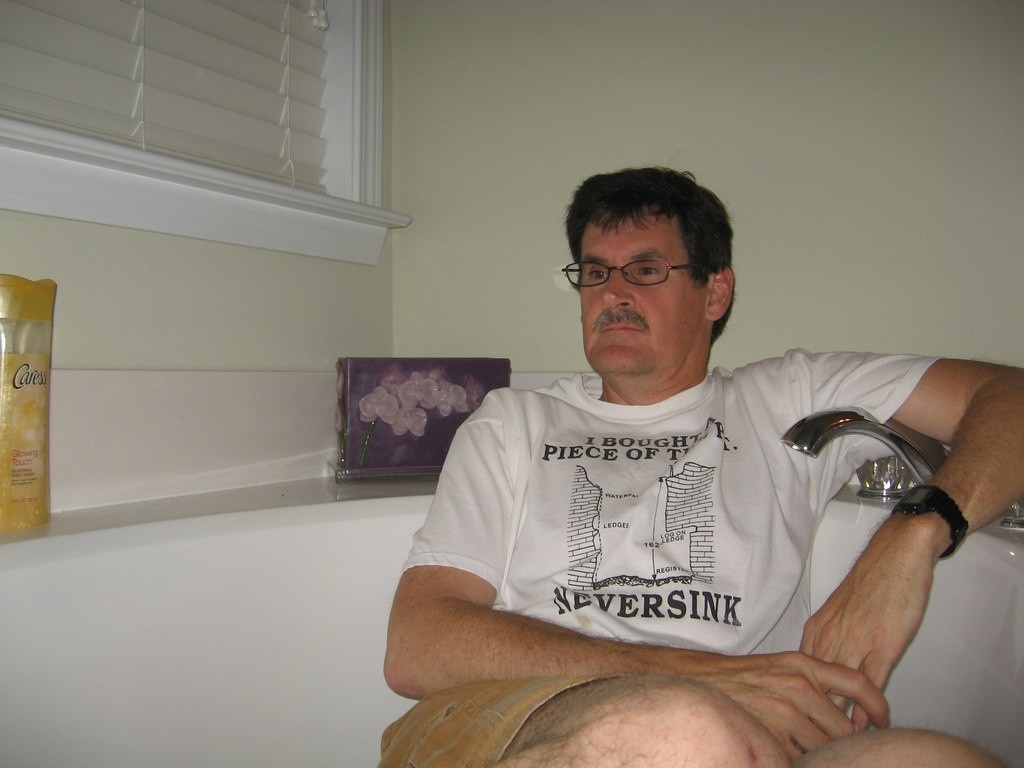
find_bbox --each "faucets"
[780,402,950,485]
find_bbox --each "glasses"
[561,258,704,288]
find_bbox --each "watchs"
[890,484,972,558]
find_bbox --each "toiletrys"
[0,273,58,535]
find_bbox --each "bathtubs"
[0,472,1024,768]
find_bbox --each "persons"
[377,165,1024,767]
